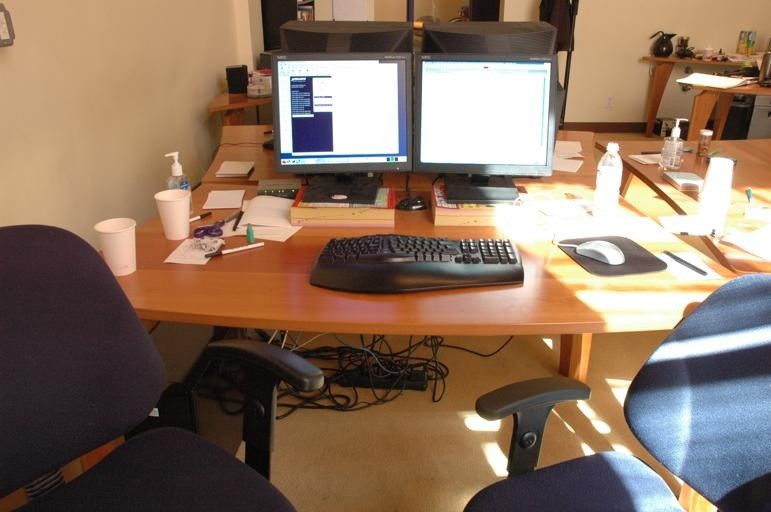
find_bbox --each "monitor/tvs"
[421,19,558,54]
[413,54,559,203]
[270,51,415,206]
[278,20,414,54]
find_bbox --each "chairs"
[460,272,769,509]
[0,224,327,511]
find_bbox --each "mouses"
[575,240,626,266]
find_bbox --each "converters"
[350,367,429,392]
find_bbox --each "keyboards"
[310,233,525,293]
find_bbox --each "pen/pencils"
[205,242,264,258]
[247,223,254,243]
[664,249,708,275]
[264,130,274,134]
[233,211,243,231]
[642,152,662,154]
[189,212,211,222]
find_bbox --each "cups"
[702,48,713,61]
[153,189,190,240]
[697,156,733,230]
[296,6,313,24]
[94,218,136,277]
[255,75,272,96]
[697,129,713,156]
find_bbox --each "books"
[291,185,396,227]
[432,181,524,225]
[214,160,255,177]
[256,177,300,197]
[677,72,758,89]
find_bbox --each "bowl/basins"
[246,85,265,98]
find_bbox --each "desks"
[639,49,771,140]
[92,182,739,388]
[201,123,597,187]
[208,88,275,124]
[599,138,770,197]
[668,175,770,276]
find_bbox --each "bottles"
[736,31,756,56]
[592,142,623,215]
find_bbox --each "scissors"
[194,212,239,238]
